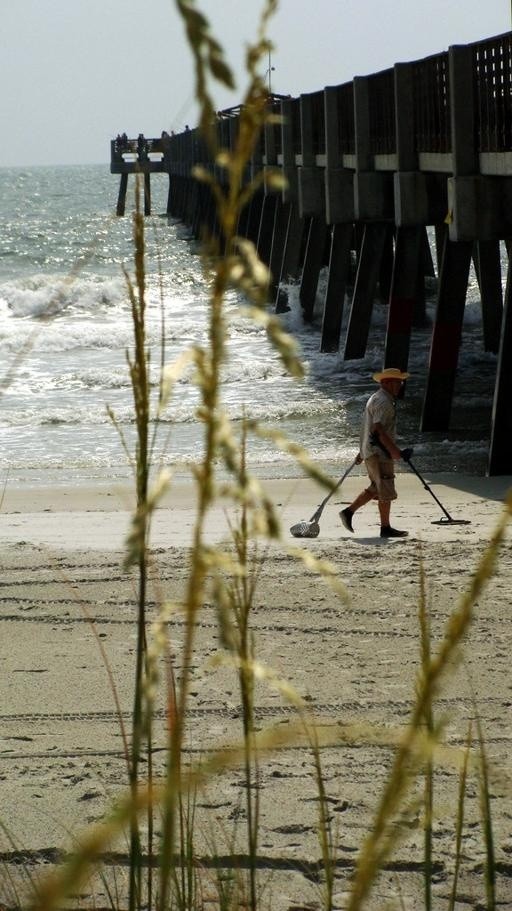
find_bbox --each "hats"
[373,368,409,382]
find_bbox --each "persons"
[184,125,190,132]
[116,132,127,152]
[339,368,411,537]
[138,133,146,153]
[212,110,224,124]
[161,131,174,139]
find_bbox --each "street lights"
[264,67,274,84]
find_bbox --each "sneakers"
[340,508,354,533]
[381,526,408,537]
